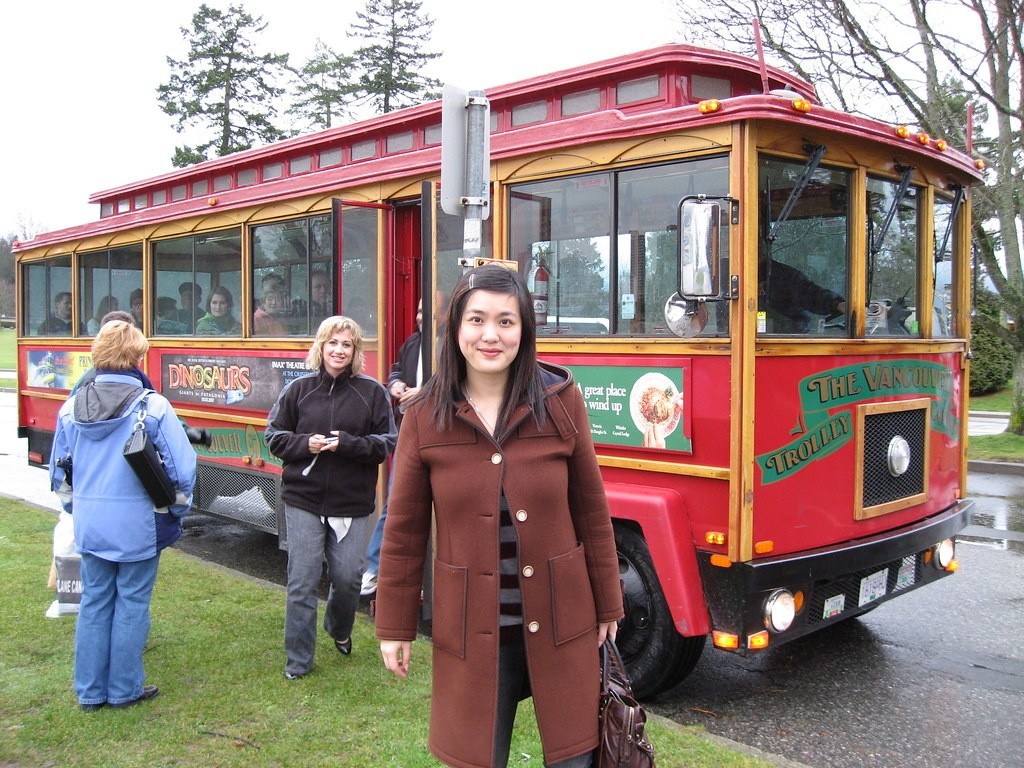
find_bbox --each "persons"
[360,291,449,593]
[38,292,87,335]
[262,273,306,336]
[267,316,398,676]
[47,320,196,706]
[88,293,118,336]
[292,269,331,336]
[254,283,293,335]
[129,289,143,333]
[374,266,625,768]
[178,282,206,321]
[158,297,193,333]
[71,312,211,450]
[197,286,242,334]
[758,221,846,334]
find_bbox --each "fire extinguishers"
[532,246,556,326]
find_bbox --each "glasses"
[132,299,143,305]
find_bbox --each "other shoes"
[358,572,378,594]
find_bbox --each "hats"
[178,282,202,295]
[130,288,143,307]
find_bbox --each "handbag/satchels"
[123,395,177,509]
[47,510,82,616]
[591,632,656,768]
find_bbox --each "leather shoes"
[109,685,160,709]
[334,636,352,655]
[81,701,106,712]
[286,671,299,680]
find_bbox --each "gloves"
[183,426,213,448]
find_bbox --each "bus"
[11,16,985,702]
